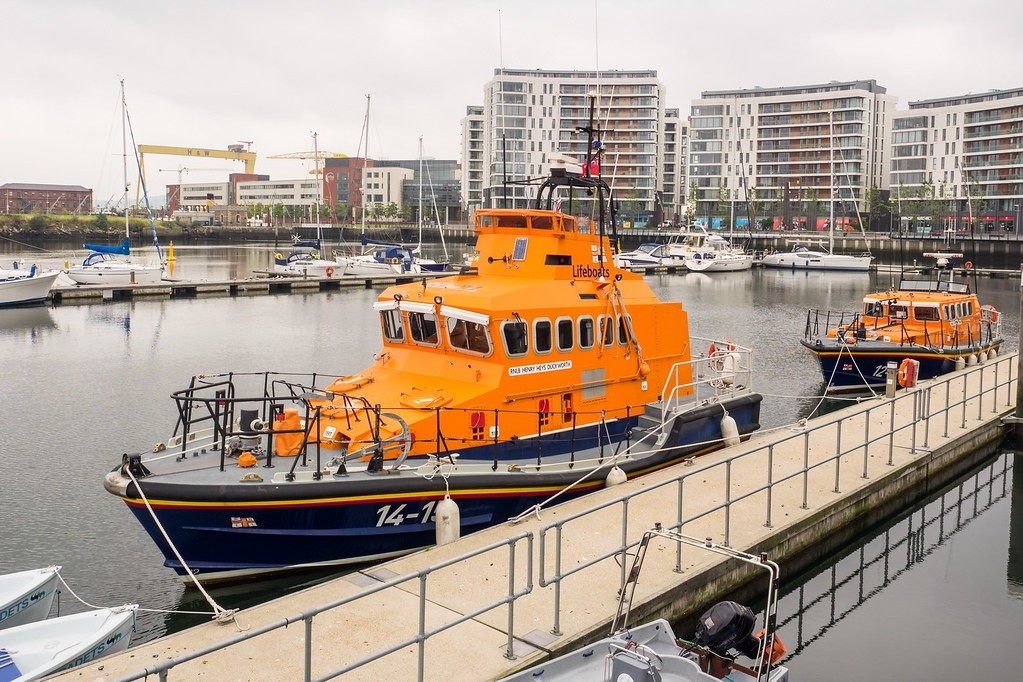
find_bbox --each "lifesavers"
[709,338,736,357]
[325,266,334,274]
[391,257,398,264]
[897,358,910,387]
[965,261,973,268]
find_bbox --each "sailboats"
[64,80,168,288]
[273,132,350,280]
[396,137,451,272]
[761,111,875,270]
[613,113,754,272]
[335,96,406,277]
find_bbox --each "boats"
[798,251,1005,404]
[0,260,64,308]
[0,603,143,681]
[489,525,792,682]
[102,92,763,581]
[0,566,62,632]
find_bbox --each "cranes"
[266,132,347,176]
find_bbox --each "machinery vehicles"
[136,140,256,214]
